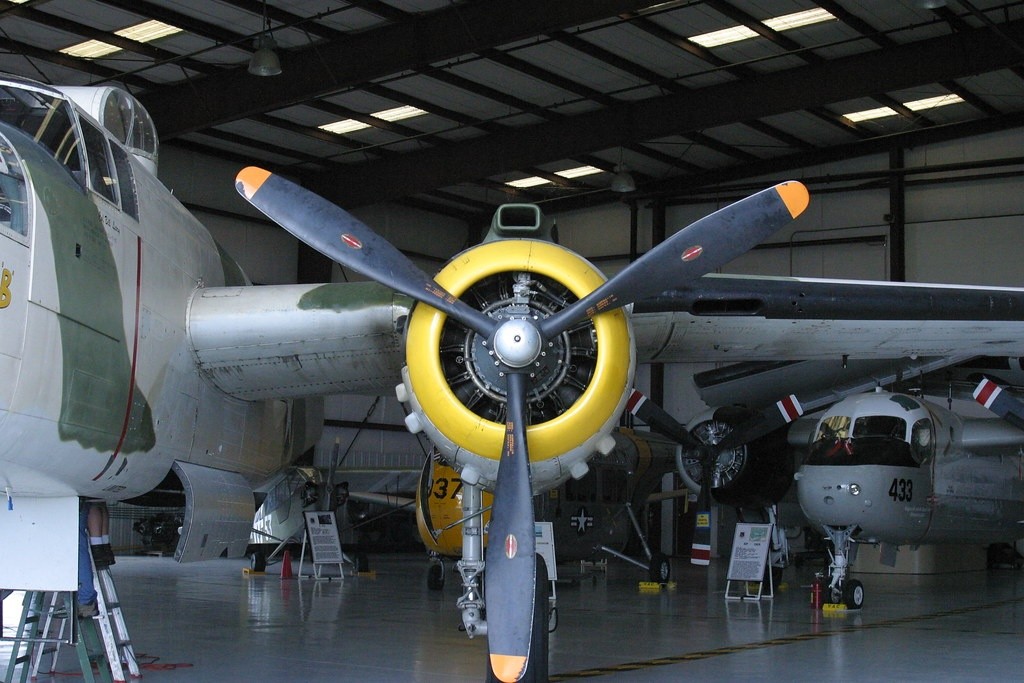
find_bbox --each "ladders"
[5,529,141,683]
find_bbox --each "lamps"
[611,146,635,192]
[248,16,282,76]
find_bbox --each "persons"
[87,501,117,568]
[66,496,100,617]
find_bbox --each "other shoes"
[90,545,108,570]
[102,543,115,565]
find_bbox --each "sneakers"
[52,600,99,619]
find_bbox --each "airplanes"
[0,65,1024,683]
